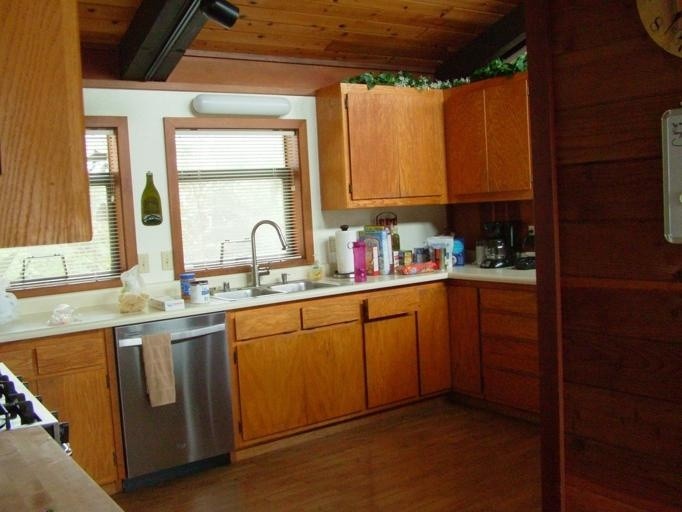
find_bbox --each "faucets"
[251,219,290,288]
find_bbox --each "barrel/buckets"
[428,237,454,275]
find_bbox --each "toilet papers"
[335,229,357,275]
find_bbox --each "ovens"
[112,312,235,481]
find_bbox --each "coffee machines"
[479,219,518,270]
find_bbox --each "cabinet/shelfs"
[445,69,534,206]
[0,327,128,503]
[224,295,363,460]
[448,271,540,423]
[364,278,449,424]
[315,82,447,213]
[0,0,94,249]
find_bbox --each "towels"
[140,329,178,411]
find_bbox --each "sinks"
[266,279,342,294]
[209,285,285,302]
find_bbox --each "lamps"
[195,0,243,31]
[191,92,294,117]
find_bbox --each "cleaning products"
[309,254,324,281]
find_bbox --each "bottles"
[179,273,196,303]
[141,171,163,225]
[475,231,486,266]
[335,211,401,283]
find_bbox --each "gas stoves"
[1,359,72,457]
